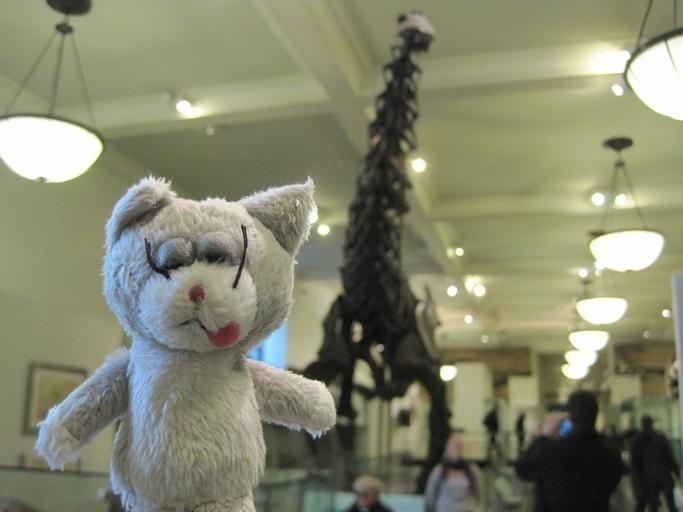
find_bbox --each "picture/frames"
[20,359,87,440]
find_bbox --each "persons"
[349,474,394,512]
[424,436,488,511]
[482,388,683,512]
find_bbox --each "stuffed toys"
[34,172,337,511]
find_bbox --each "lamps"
[620,1,683,123]
[557,280,611,382]
[584,135,667,275]
[571,230,633,327]
[0,0,109,185]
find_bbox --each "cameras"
[558,416,572,437]
